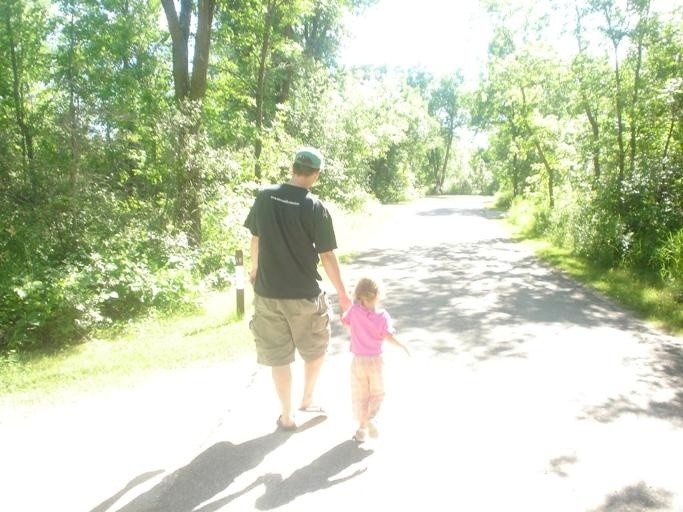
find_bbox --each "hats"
[293,148,327,174]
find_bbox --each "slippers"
[277,407,297,430]
[299,403,329,415]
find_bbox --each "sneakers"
[365,418,379,438]
[355,426,368,442]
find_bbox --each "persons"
[338,278,409,443]
[244,150,353,431]
